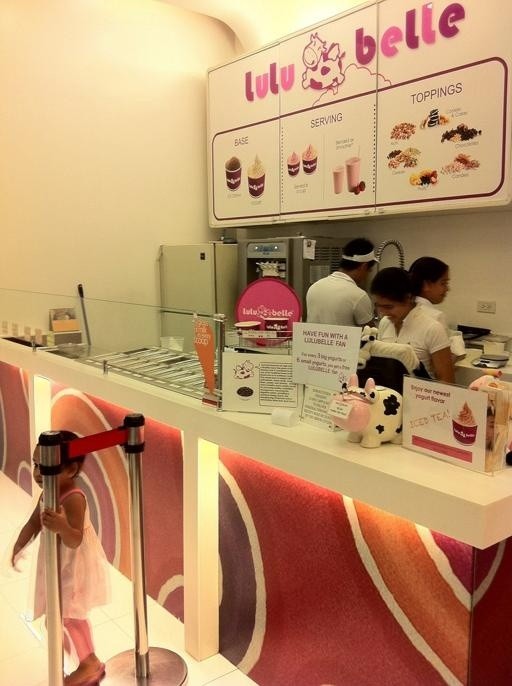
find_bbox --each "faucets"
[375,238,404,271]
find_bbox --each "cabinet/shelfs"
[206,0,512,228]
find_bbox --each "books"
[480,380,512,472]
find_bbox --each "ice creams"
[302,144,316,161]
[225,156,241,171]
[248,154,264,179]
[287,151,300,165]
[452,400,476,427]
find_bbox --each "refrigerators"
[159,245,238,357]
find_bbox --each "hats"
[341,249,381,265]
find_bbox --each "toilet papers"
[270,408,299,427]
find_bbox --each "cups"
[233,317,288,337]
[225,163,242,190]
[303,156,317,171]
[333,159,361,194]
[453,417,478,447]
[246,166,265,199]
[286,160,299,175]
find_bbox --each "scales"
[470,353,509,369]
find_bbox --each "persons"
[409,256,466,363]
[307,238,376,348]
[370,267,455,384]
[11,431,105,686]
